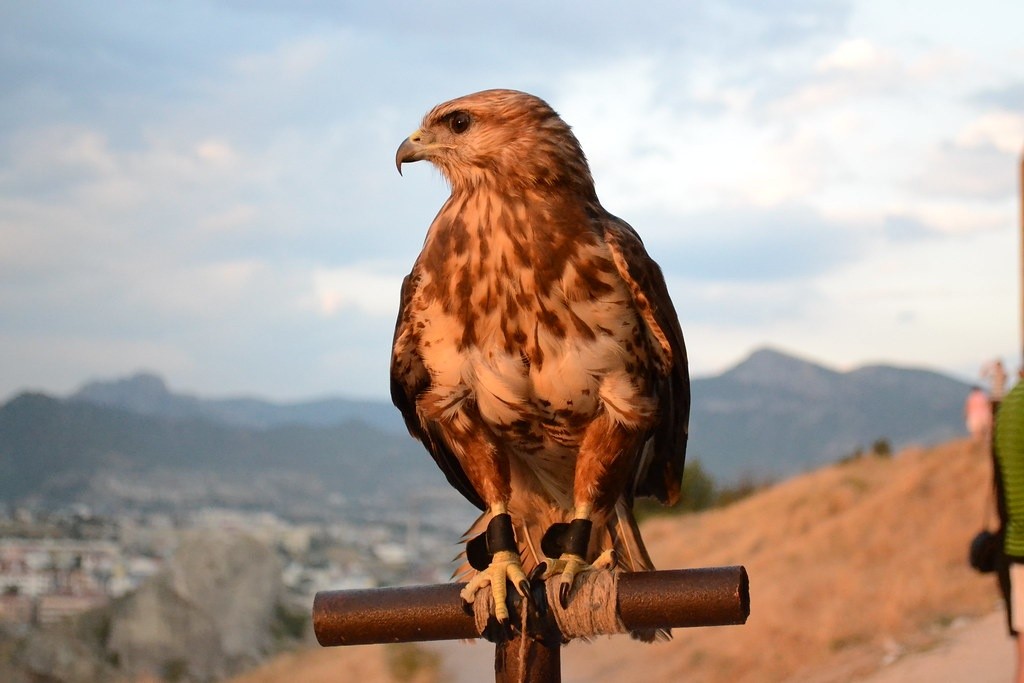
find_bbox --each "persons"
[964,362,1024,683]
